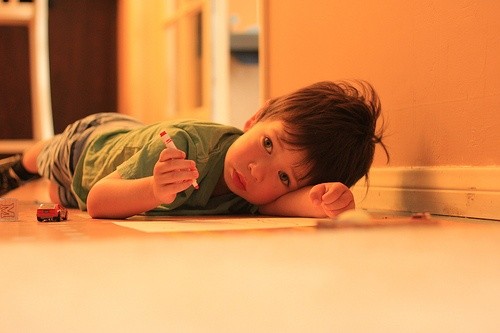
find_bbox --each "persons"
[23,80,389,220]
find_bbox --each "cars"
[36,202,68,222]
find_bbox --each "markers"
[160,131,199,190]
